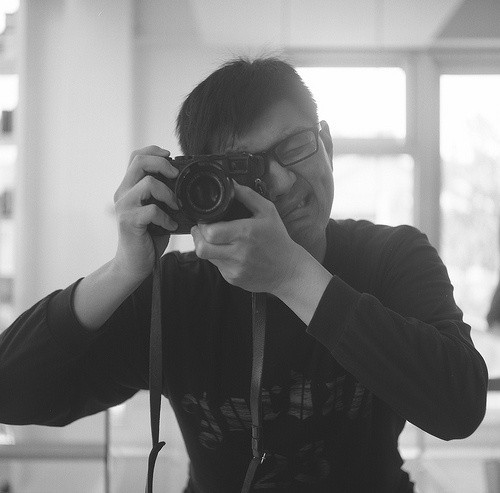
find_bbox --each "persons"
[2,54,490,493]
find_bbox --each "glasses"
[246,122,321,178]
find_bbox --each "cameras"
[141,152,255,237]
